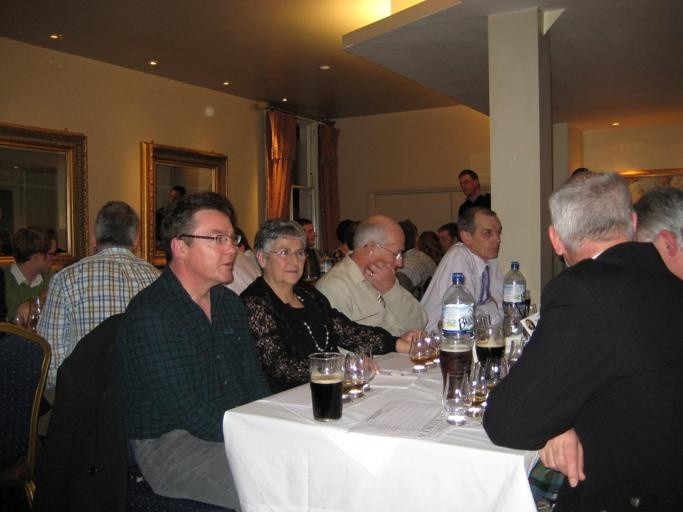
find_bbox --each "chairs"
[1,317,55,512]
[54,311,238,512]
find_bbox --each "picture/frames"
[140,138,231,271]
[0,120,90,270]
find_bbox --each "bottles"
[441,273,474,346]
[525,290,536,316]
[501,260,526,334]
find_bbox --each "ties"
[478,267,491,305]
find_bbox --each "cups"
[410,329,440,372]
[10,295,45,334]
[308,353,344,421]
[337,344,377,404]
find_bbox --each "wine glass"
[437,324,525,428]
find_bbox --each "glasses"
[178,233,241,245]
[363,242,403,261]
[269,249,309,260]
